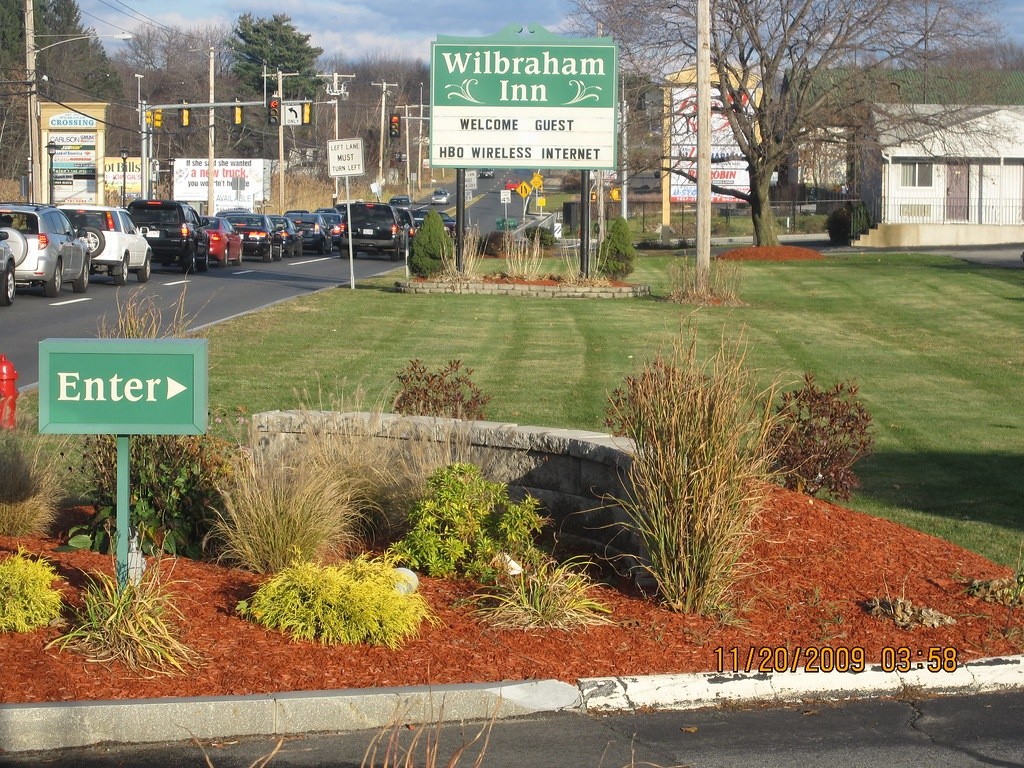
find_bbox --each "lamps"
[936,166,941,177]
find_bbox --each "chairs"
[26,215,38,234]
[414,213,419,218]
[276,223,284,229]
[373,213,385,219]
[358,209,369,215]
[145,212,161,223]
[2,215,13,227]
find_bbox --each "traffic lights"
[144,110,151,124]
[590,192,597,201]
[389,113,401,138]
[268,97,282,126]
[153,109,162,128]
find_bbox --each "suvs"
[339,200,410,263]
[0,199,92,301]
[55,204,153,288]
[124,198,212,276]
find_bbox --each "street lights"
[43,141,62,205]
[26,32,134,208]
[119,147,132,210]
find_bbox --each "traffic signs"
[284,105,302,125]
[37,337,208,435]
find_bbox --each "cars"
[478,168,496,179]
[212,186,455,265]
[197,215,244,270]
[503,177,522,192]
[0,230,16,307]
